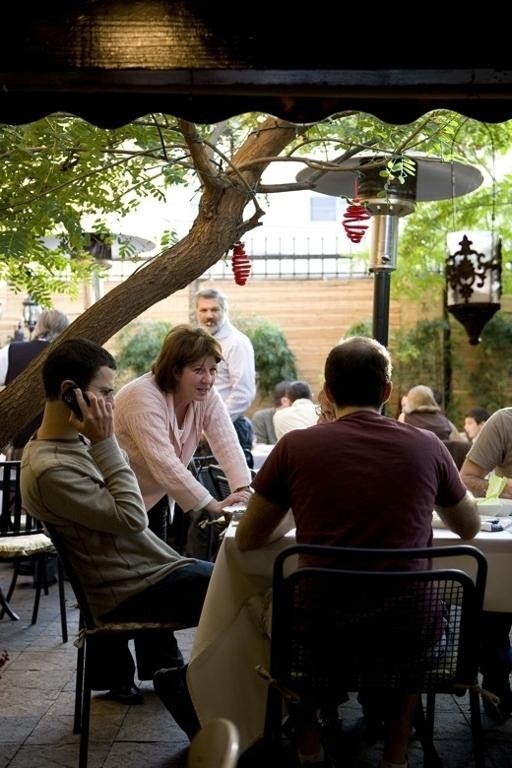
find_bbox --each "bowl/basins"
[473,498,512,516]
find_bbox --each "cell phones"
[62,385,88,418]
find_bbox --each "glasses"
[90,384,114,399]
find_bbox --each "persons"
[0,310,68,483]
[163,286,258,556]
[454,405,512,722]
[233,336,482,767]
[251,375,488,446]
[18,336,215,742]
[92,321,252,707]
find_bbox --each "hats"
[402,385,440,413]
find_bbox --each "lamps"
[444,118,502,345]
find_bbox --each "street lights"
[298,148,484,353]
[442,226,501,408]
[23,297,47,338]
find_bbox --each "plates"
[221,505,248,514]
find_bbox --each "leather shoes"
[112,684,144,704]
